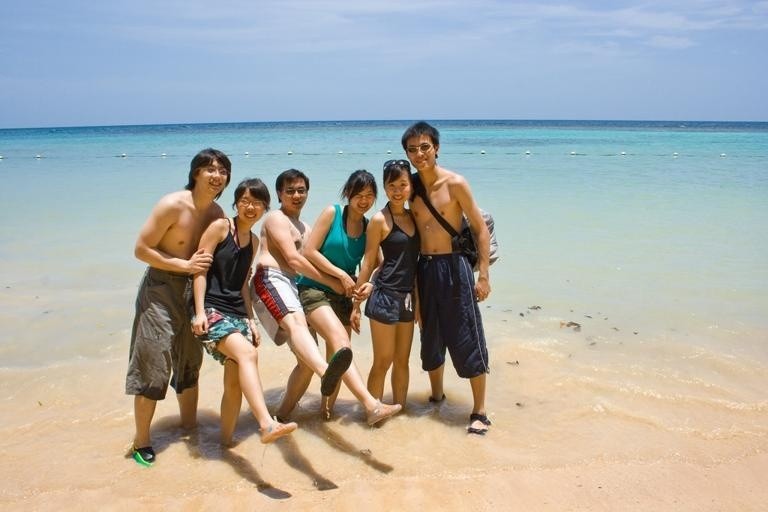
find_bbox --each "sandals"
[428,393,447,412]
[321,347,354,397]
[367,400,401,425]
[261,421,299,442]
[467,412,492,438]
[130,444,157,469]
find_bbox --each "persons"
[125,146,232,466]
[402,121,492,434]
[250,168,352,415]
[351,159,421,408]
[191,178,298,448]
[297,170,402,425]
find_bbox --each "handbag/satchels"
[462,205,500,272]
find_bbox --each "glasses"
[285,187,308,194]
[383,159,410,172]
[238,198,266,209]
[406,143,432,153]
